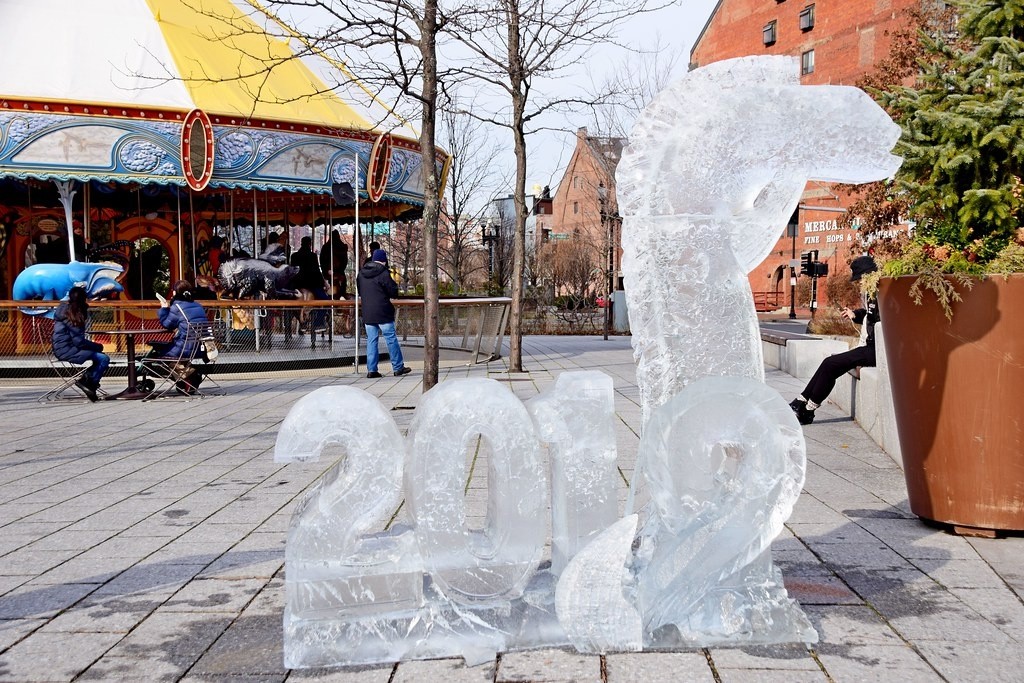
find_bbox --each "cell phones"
[835,302,844,312]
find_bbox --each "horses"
[615,54,904,501]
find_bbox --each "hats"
[372,249,388,262]
[849,257,877,282]
[73,220,83,230]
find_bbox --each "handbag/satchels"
[200,340,219,363]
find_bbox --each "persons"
[209,230,380,332]
[51,286,111,403]
[358,250,411,379]
[789,256,880,426]
[157,280,210,394]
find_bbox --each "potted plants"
[859,239,1024,538]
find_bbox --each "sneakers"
[790,400,806,411]
[794,405,815,425]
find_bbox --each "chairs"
[35,322,109,404]
[140,321,229,401]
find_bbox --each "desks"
[87,329,173,401]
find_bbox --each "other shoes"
[394,367,411,376]
[82,388,98,402]
[367,372,383,378]
[76,378,95,395]
[185,369,199,394]
